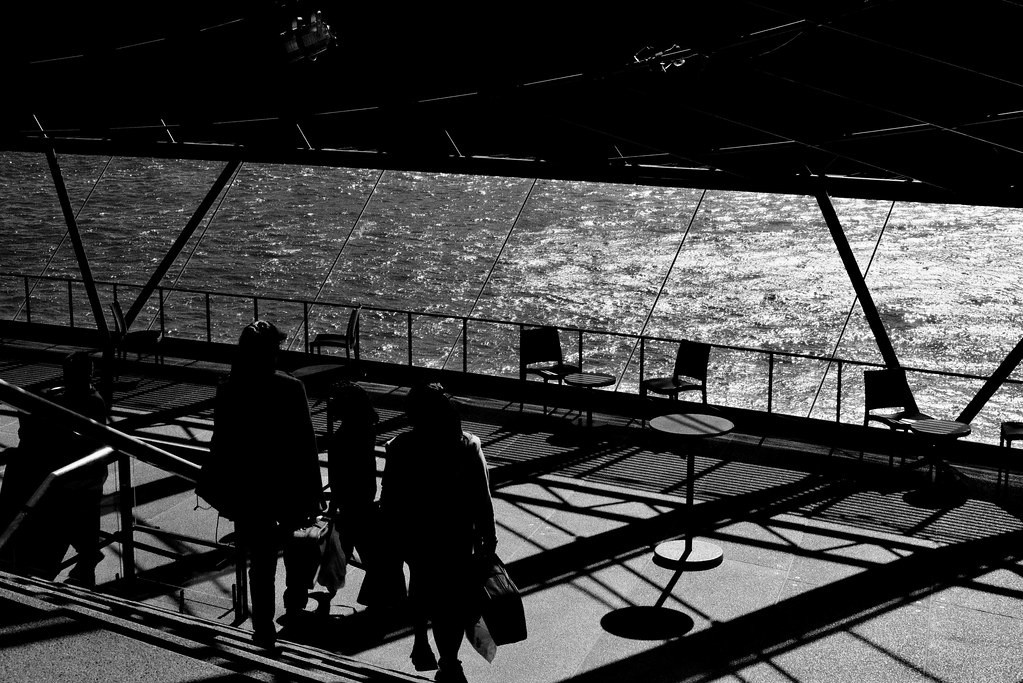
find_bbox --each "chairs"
[859,368,936,472]
[640,338,712,430]
[108,300,165,382]
[309,302,368,382]
[519,325,583,417]
[996,422,1023,499]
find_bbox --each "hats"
[239,321,287,350]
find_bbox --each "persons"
[213,319,327,636]
[322,379,498,683]
[40,350,108,587]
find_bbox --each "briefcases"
[291,516,330,590]
[473,546,526,646]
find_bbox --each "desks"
[563,371,617,436]
[649,414,736,569]
[910,418,974,493]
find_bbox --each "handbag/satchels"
[318,523,346,590]
[195,435,237,525]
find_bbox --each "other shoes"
[252,628,278,645]
[436,660,467,683]
[410,644,439,672]
